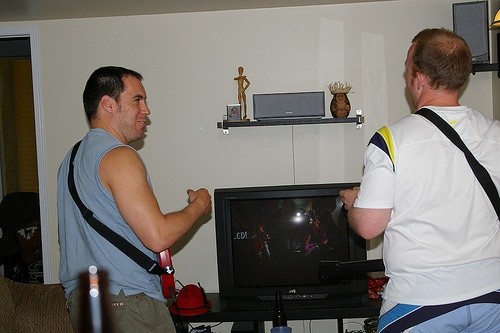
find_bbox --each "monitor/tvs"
[215,182,367,308]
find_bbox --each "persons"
[234,67,250,119]
[56,65,212,333]
[339,29,500,333]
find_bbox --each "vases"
[330,92,351,118]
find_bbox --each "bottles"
[272,289,287,333]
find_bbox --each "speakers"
[253,91,325,120]
[452,1,490,63]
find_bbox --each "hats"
[170,284,212,316]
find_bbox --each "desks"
[170,293,382,333]
